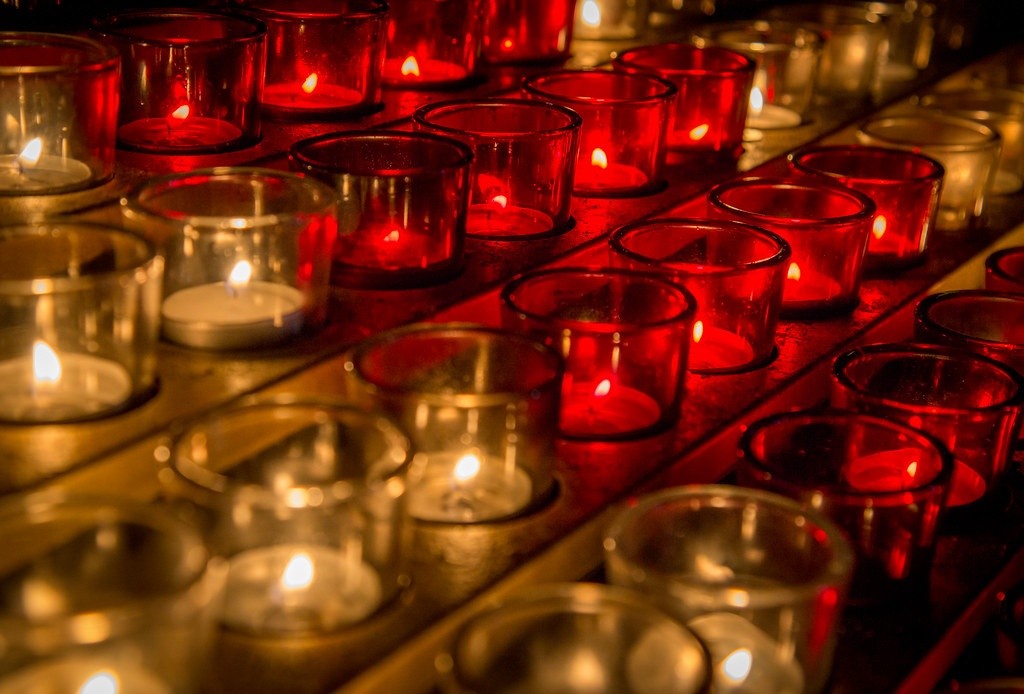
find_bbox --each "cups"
[0,0,1024,694]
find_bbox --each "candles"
[0,0,1024,694]
